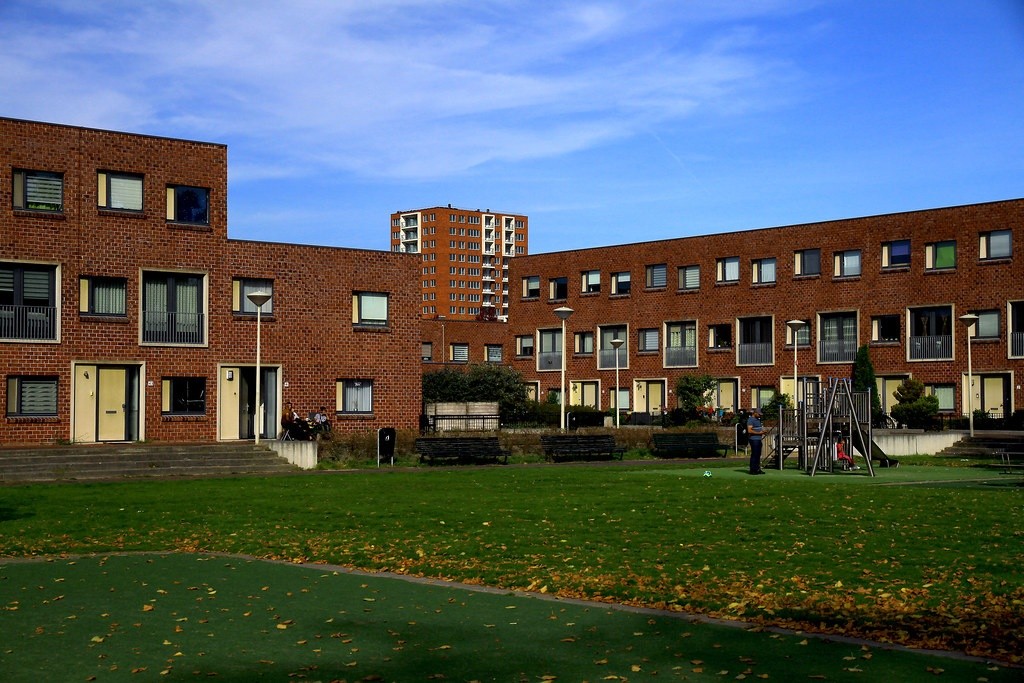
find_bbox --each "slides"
[842,430,898,468]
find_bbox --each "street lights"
[554,306,574,432]
[957,315,979,437]
[611,338,625,430]
[249,291,271,443]
[785,319,806,435]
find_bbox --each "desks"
[987,452,1024,474]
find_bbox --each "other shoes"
[759,470,766,474]
[751,470,758,475]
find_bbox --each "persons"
[747,408,769,475]
[308,406,330,440]
[281,402,307,440]
[837,438,860,471]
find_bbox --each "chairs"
[280,412,331,443]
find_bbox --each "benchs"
[654,433,730,458]
[415,437,512,468]
[541,435,627,462]
[991,464,1024,468]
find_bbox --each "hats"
[755,408,763,415]
